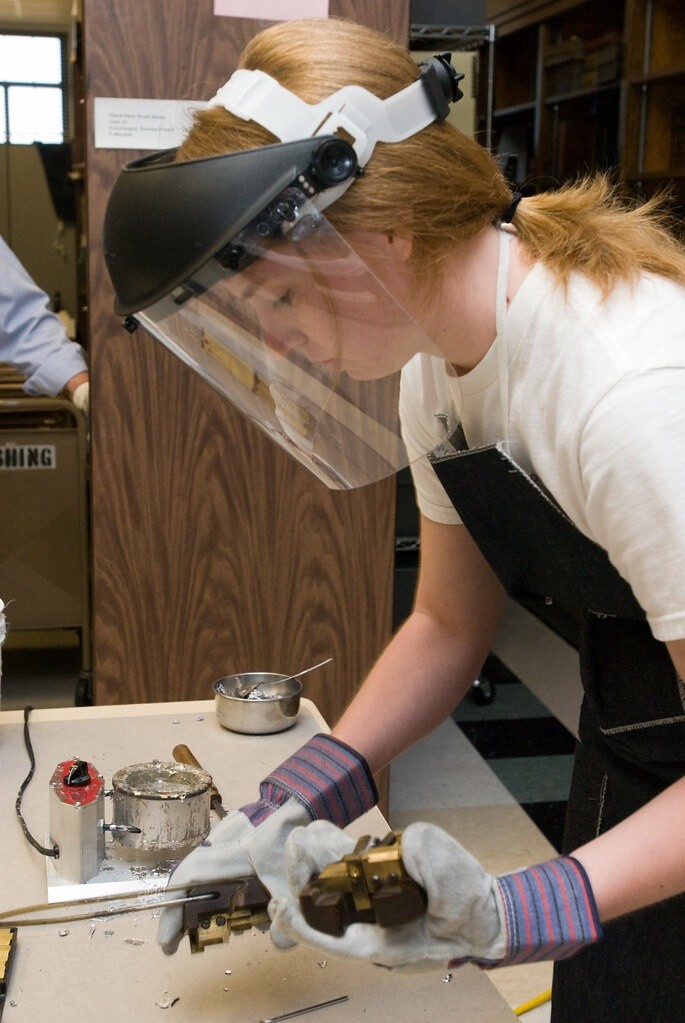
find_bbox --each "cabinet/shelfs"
[476,0,684,242]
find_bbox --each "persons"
[101,16,685,1023]
[0,234,89,415]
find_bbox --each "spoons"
[242,657,334,699]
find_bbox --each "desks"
[0,696,522,1023]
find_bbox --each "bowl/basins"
[212,671,304,735]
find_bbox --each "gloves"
[154,731,379,956]
[249,819,604,971]
